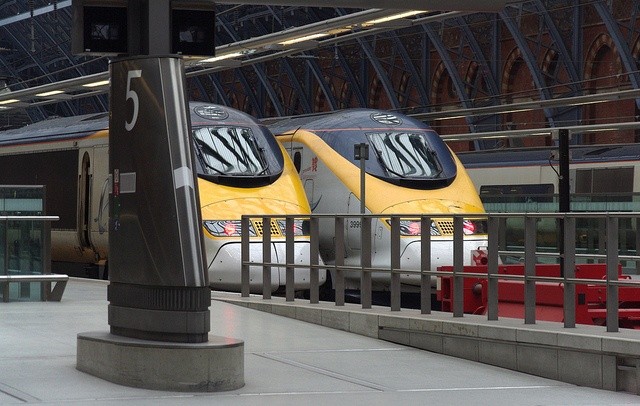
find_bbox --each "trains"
[259,107,504,294]
[0,101,328,295]
[453,144,640,266]
